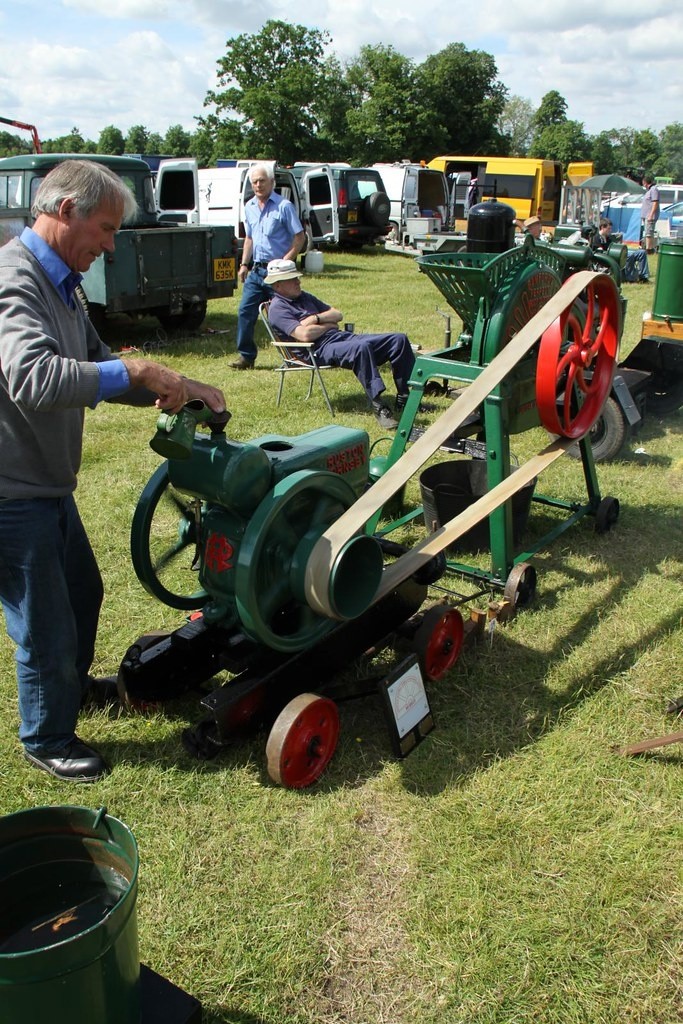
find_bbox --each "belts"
[254,261,268,269]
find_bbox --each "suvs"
[279,164,394,250]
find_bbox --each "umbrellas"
[580,173,647,217]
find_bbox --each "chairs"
[259,299,342,418]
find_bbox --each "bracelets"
[240,263,249,267]
[314,313,320,324]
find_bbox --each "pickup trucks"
[0,153,239,331]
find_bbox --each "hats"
[264,259,303,284]
[523,216,539,227]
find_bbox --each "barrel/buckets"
[0,807,140,1023]
[368,437,407,517]
[305,249,324,273]
[419,453,539,553]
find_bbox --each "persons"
[264,259,428,430]
[593,219,653,283]
[0,159,229,781]
[525,215,543,240]
[227,163,305,368]
[640,176,661,254]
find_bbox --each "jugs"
[149,398,213,460]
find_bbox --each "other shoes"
[227,357,255,371]
[394,394,430,414]
[639,277,650,284]
[646,248,655,255]
[373,404,399,430]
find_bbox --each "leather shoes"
[25,734,111,783]
[81,675,121,713]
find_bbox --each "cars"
[594,184,683,241]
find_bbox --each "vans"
[151,161,340,264]
[420,155,594,233]
[0,156,200,227]
[358,163,472,242]
